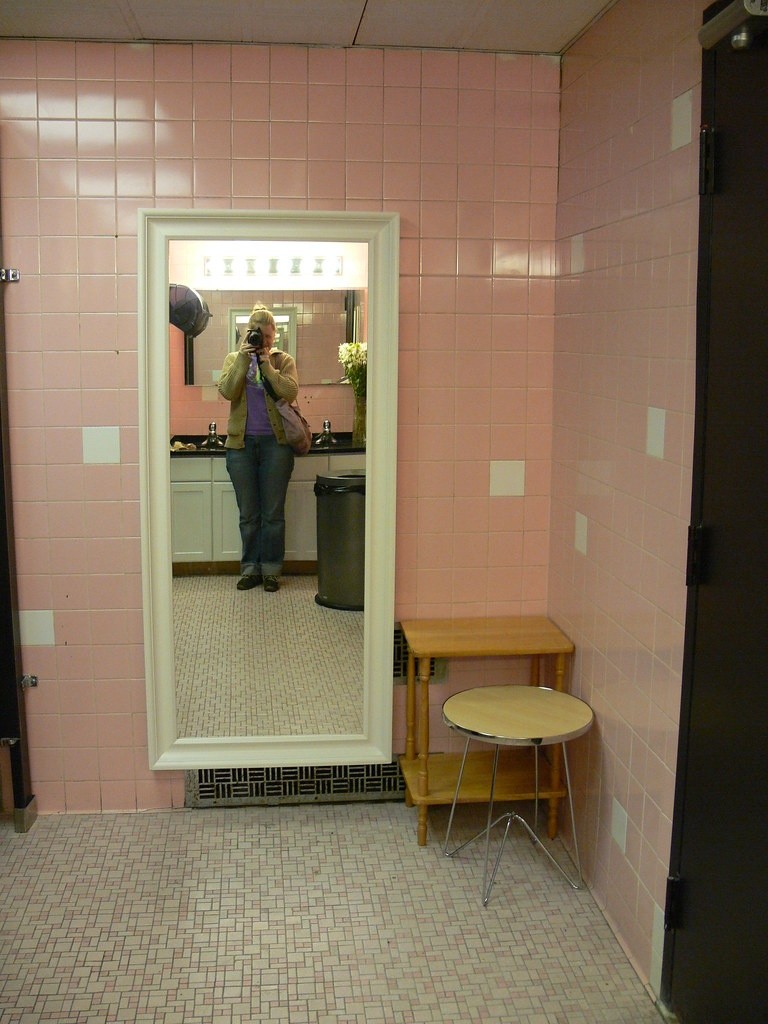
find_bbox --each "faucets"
[201,421,226,447]
[315,420,337,444]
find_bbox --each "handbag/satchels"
[275,396,312,454]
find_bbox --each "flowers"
[337,342,367,395]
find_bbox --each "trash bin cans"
[313,468,366,611]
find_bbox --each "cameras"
[246,329,264,353]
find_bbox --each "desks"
[441,685,594,907]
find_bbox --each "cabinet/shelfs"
[398,616,574,847]
[169,455,369,577]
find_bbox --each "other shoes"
[264,574,278,591]
[237,574,262,591]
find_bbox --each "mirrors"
[136,208,401,773]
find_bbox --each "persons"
[218,303,299,592]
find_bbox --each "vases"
[352,396,367,449]
